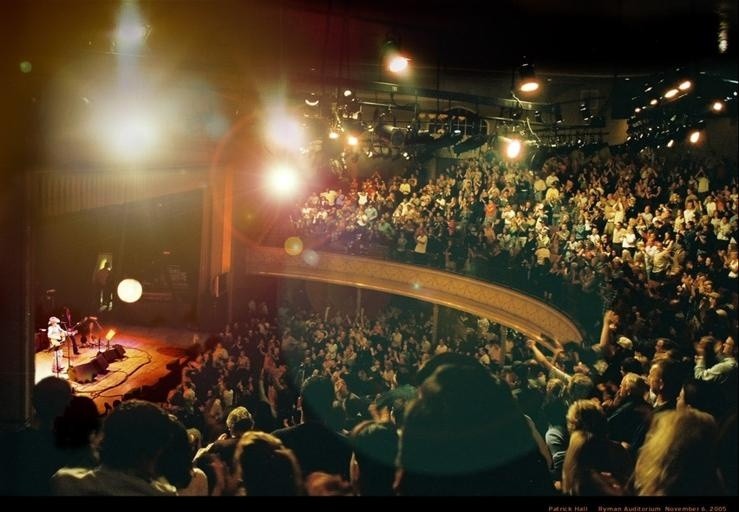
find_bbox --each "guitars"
[51,330,78,347]
[66,321,82,333]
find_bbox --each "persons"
[103,270,112,311]
[60,303,80,354]
[171,139,739,497]
[48,316,78,372]
[95,262,111,307]
[19,375,171,497]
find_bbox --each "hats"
[48,317,61,325]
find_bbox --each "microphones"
[60,321,68,324]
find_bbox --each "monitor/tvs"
[213,272,228,298]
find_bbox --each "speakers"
[166,357,179,370]
[71,343,126,385]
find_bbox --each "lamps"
[516,50,540,92]
[378,1,410,73]
